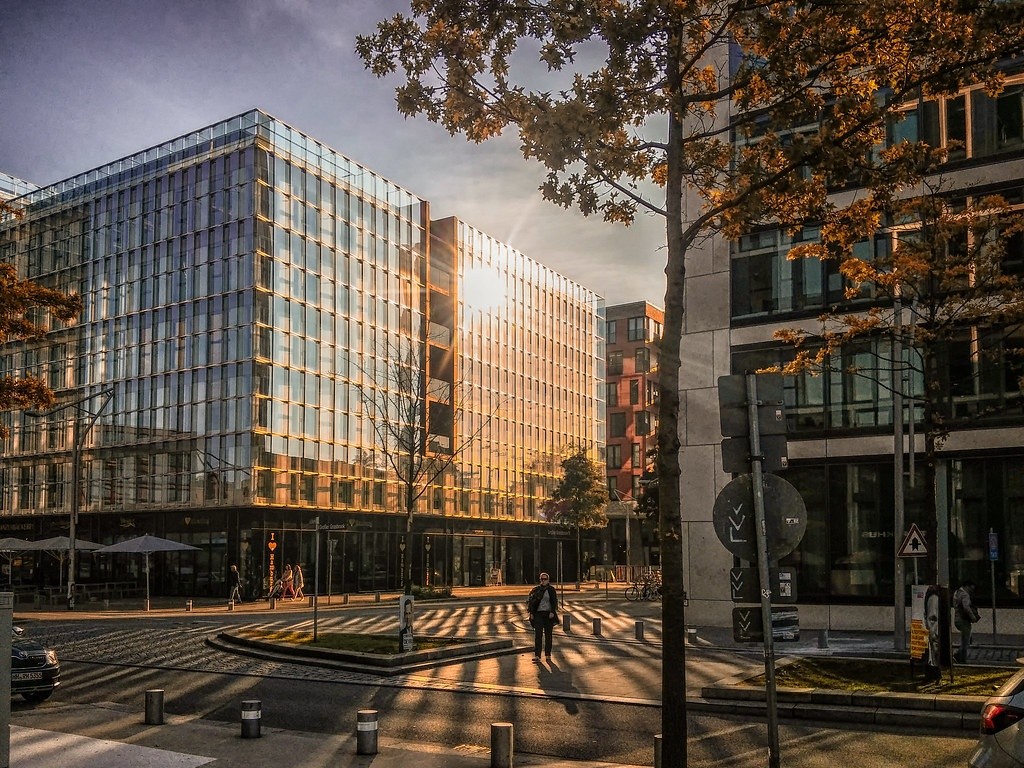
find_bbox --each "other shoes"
[236,601,242,604]
[290,598,294,602]
[545,651,551,656]
[301,598,304,601]
[532,658,540,661]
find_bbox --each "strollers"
[262,579,284,602]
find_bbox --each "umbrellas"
[91,533,204,612]
[0,534,109,592]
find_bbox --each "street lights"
[25,385,116,610]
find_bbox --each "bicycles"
[622,577,661,602]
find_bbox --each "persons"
[400,599,413,653]
[924,585,939,667]
[227,565,243,604]
[952,580,977,664]
[279,564,304,602]
[526,573,560,661]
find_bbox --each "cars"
[965,658,1024,768]
[11,626,60,704]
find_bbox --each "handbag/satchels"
[958,604,981,623]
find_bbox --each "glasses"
[541,578,548,581]
